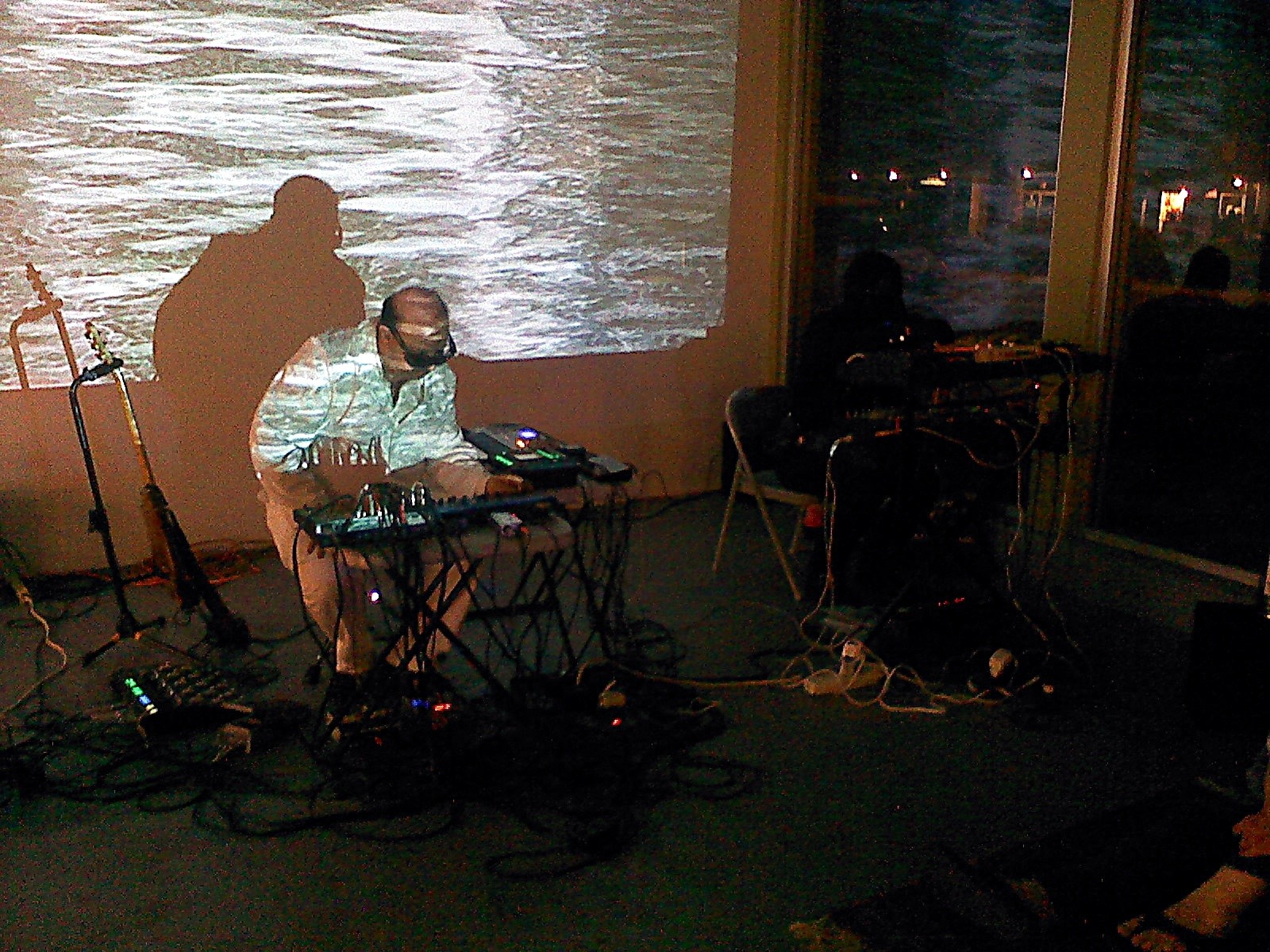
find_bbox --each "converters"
[989,648,1012,668]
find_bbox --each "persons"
[1087,223,1270,579]
[1114,742,1270,952]
[803,249,1005,534]
[249,287,523,695]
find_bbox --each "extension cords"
[803,670,883,694]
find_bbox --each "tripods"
[68,373,175,668]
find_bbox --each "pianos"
[814,343,1114,405]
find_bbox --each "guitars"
[83,320,203,608]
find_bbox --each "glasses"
[388,321,456,367]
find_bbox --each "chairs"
[711,382,822,602]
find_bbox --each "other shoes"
[326,674,358,703]
[384,662,426,698]
